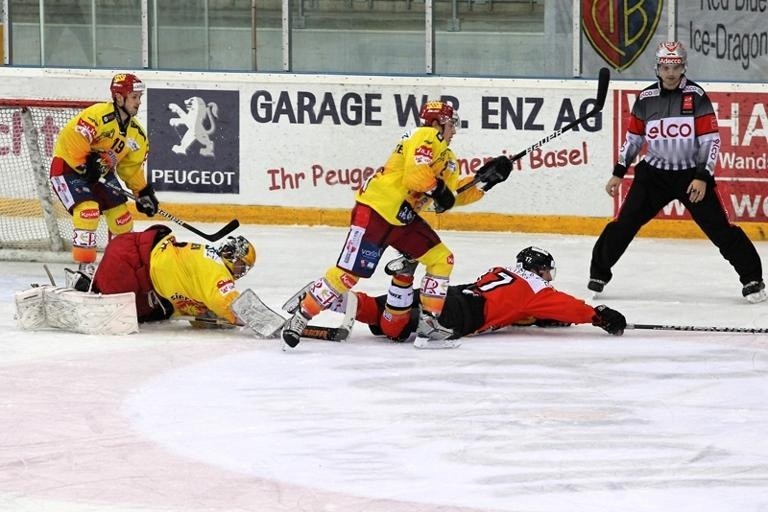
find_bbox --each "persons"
[64,225,256,330]
[589,41,766,298]
[48,73,158,292]
[281,101,511,349]
[283,245,627,342]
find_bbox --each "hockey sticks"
[167,290,358,340]
[434,68,611,210]
[99,177,240,242]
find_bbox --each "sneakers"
[384,255,418,276]
[416,303,460,341]
[283,308,312,348]
[287,293,308,314]
[588,279,607,292]
[742,278,765,297]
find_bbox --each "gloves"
[135,185,159,217]
[424,177,455,214]
[86,153,102,185]
[592,304,627,336]
[474,156,513,192]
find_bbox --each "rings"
[691,189,697,193]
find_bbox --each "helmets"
[656,41,689,70]
[516,245,557,282]
[216,235,256,281]
[110,74,145,98]
[419,102,461,128]
